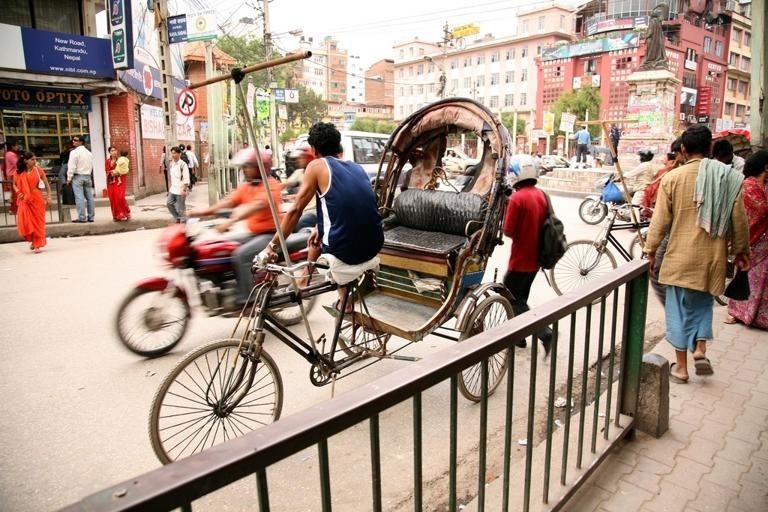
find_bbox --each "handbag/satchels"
[35,166,46,189]
[724,266,750,300]
[180,165,197,189]
[602,182,623,202]
[60,183,76,205]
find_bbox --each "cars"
[536,154,592,175]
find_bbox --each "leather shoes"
[542,333,553,356]
[71,220,86,223]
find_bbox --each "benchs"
[372,187,481,305]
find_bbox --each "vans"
[298,129,410,187]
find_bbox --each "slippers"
[332,299,353,315]
[274,282,312,300]
[694,359,713,375]
[668,362,687,384]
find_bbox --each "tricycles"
[148,97,515,465]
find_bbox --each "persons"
[110,149,130,187]
[55,139,74,206]
[186,150,282,306]
[447,150,454,158]
[607,120,768,385]
[157,144,209,225]
[636,7,670,71]
[66,135,96,223]
[527,122,613,178]
[104,145,132,223]
[269,132,318,231]
[501,152,558,364]
[3,138,20,214]
[12,151,53,254]
[261,120,385,316]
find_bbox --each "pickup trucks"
[440,146,482,173]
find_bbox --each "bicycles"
[551,201,648,305]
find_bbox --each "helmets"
[289,142,317,159]
[232,147,272,176]
[637,148,654,162]
[506,154,538,188]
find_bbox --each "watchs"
[269,242,280,250]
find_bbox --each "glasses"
[72,138,79,142]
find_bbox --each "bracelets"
[16,191,20,195]
[47,198,51,200]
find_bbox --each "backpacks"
[537,190,568,270]
[180,151,189,164]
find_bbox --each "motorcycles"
[117,211,317,357]
[580,172,638,224]
